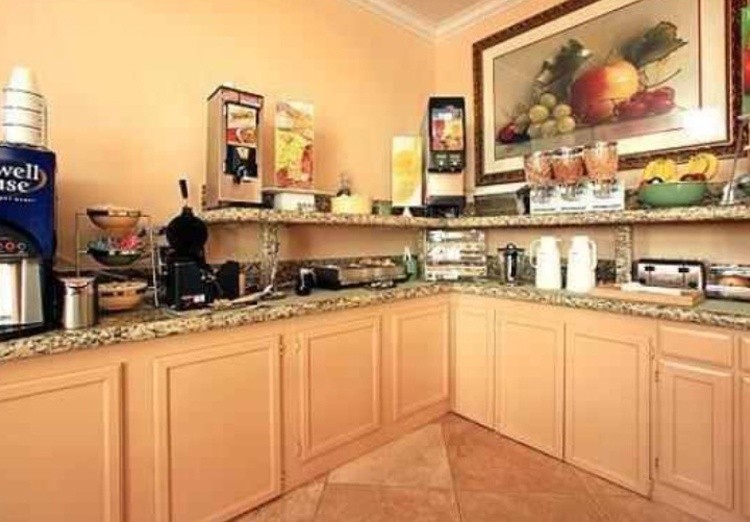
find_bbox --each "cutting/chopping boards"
[586,282,705,306]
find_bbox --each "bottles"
[396,246,418,282]
[337,174,350,196]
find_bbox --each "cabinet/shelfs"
[0,292,750,522]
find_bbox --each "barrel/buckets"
[51,274,97,329]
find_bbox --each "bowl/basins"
[639,181,707,208]
[4,66,44,145]
[329,196,373,214]
[374,197,393,214]
[409,206,430,217]
[87,207,140,232]
[97,280,148,311]
[88,241,144,267]
[391,207,405,214]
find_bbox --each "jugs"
[528,236,563,289]
[497,243,525,288]
[565,235,598,292]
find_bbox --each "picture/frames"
[473,0,747,187]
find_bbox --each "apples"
[569,46,638,121]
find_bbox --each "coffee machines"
[0,143,57,344]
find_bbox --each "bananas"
[644,156,677,181]
[684,152,718,180]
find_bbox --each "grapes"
[525,92,577,139]
[615,86,675,119]
